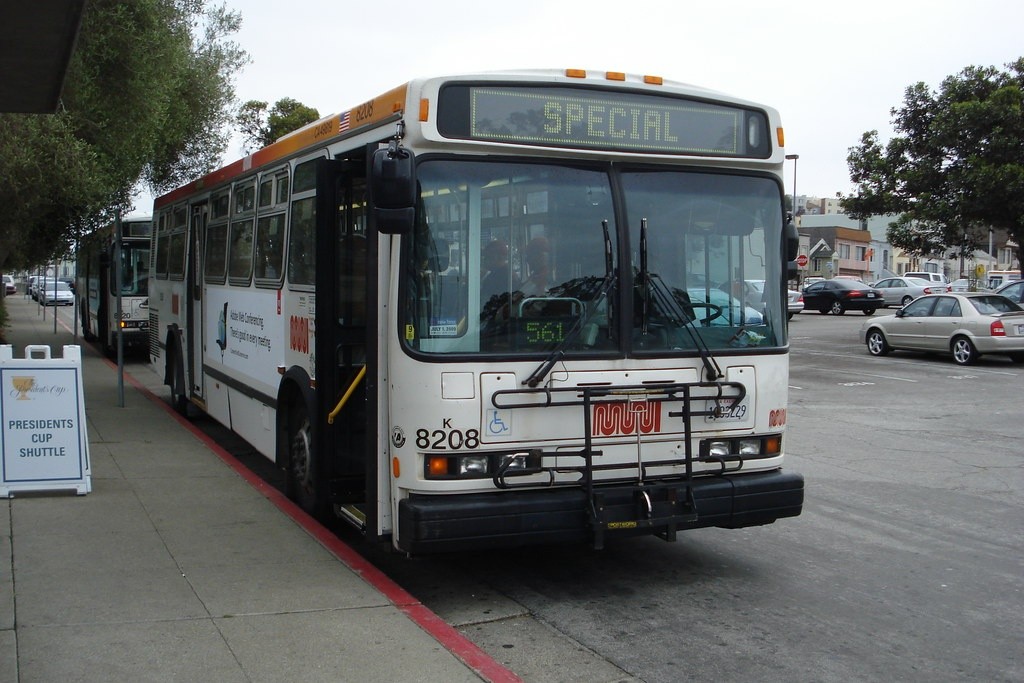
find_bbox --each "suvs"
[2,275,17,295]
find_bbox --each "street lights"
[785,154,798,223]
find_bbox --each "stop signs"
[797,254,808,266]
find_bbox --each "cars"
[27,275,75,307]
[949,278,1024,310]
[788,289,805,321]
[803,279,886,316]
[859,292,1024,366]
[869,277,953,307]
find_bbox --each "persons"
[455,235,550,330]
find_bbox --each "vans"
[903,272,948,283]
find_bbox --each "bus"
[76,218,153,360]
[148,68,804,551]
